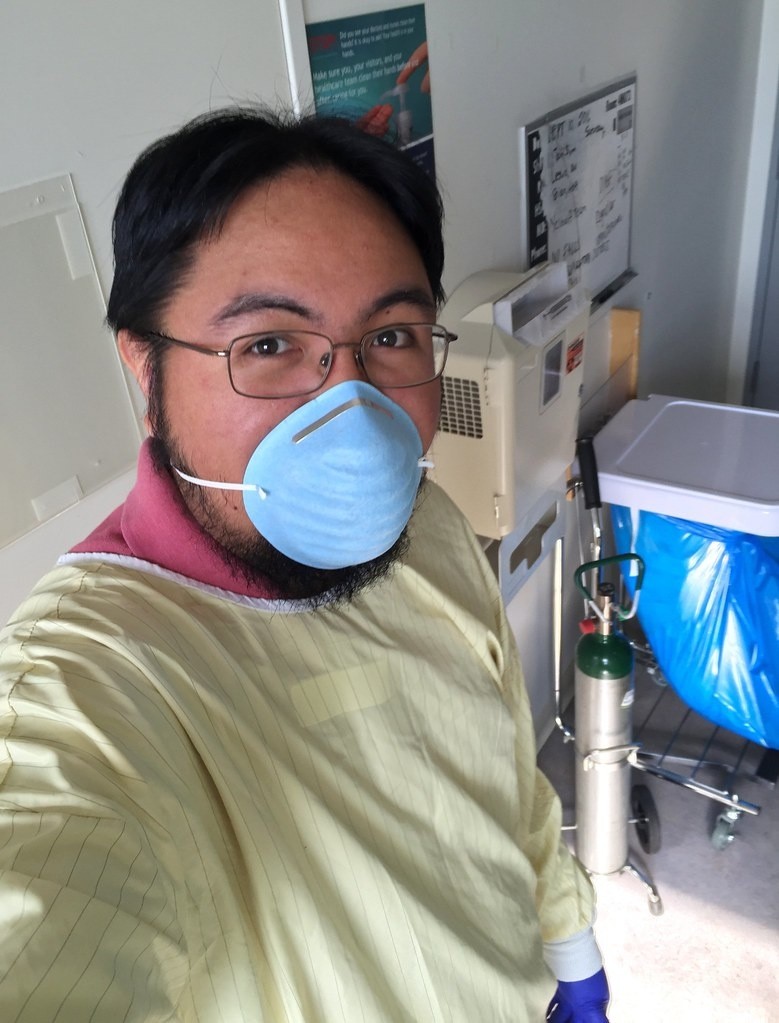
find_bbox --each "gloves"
[544,966,610,1023]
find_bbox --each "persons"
[0,100,611,1023]
[354,40,430,136]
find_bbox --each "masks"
[170,379,435,569]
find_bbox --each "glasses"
[142,323,458,400]
